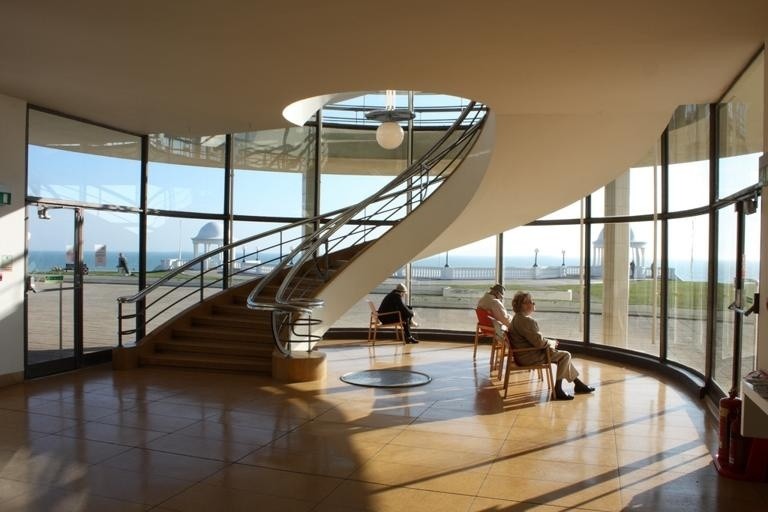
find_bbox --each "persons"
[377,282,419,344]
[477,283,513,337]
[506,291,596,401]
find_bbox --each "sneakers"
[574,385,594,393]
[551,389,574,400]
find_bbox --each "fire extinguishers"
[719,389,743,469]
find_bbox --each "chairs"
[364,298,407,348]
[474,308,559,399]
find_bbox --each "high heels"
[406,337,418,344]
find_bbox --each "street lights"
[562,250,565,266]
[532,248,539,267]
[290,245,295,264]
[255,245,260,261]
[242,246,246,262]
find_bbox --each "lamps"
[362,90,417,153]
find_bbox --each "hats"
[397,283,408,292]
[490,284,505,298]
